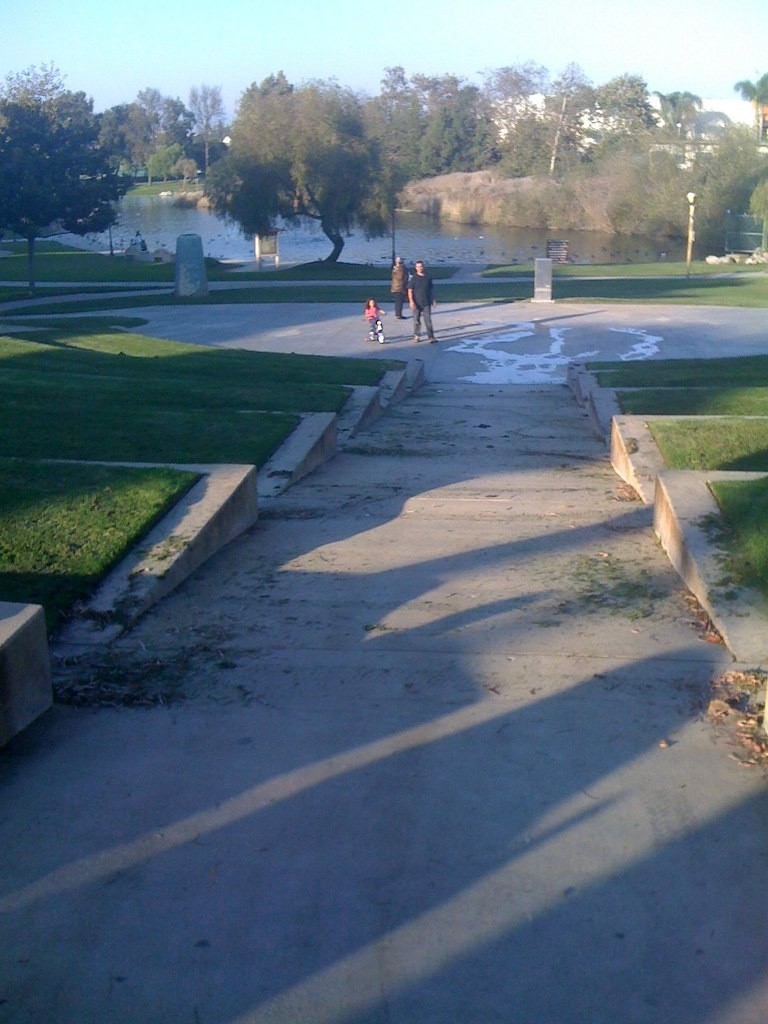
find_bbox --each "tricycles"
[364,314,385,344]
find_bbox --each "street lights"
[685,192,698,278]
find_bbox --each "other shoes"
[429,336,438,343]
[413,336,419,343]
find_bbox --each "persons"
[365,297,385,341]
[390,256,409,320]
[405,261,438,344]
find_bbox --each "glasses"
[398,261,403,264]
